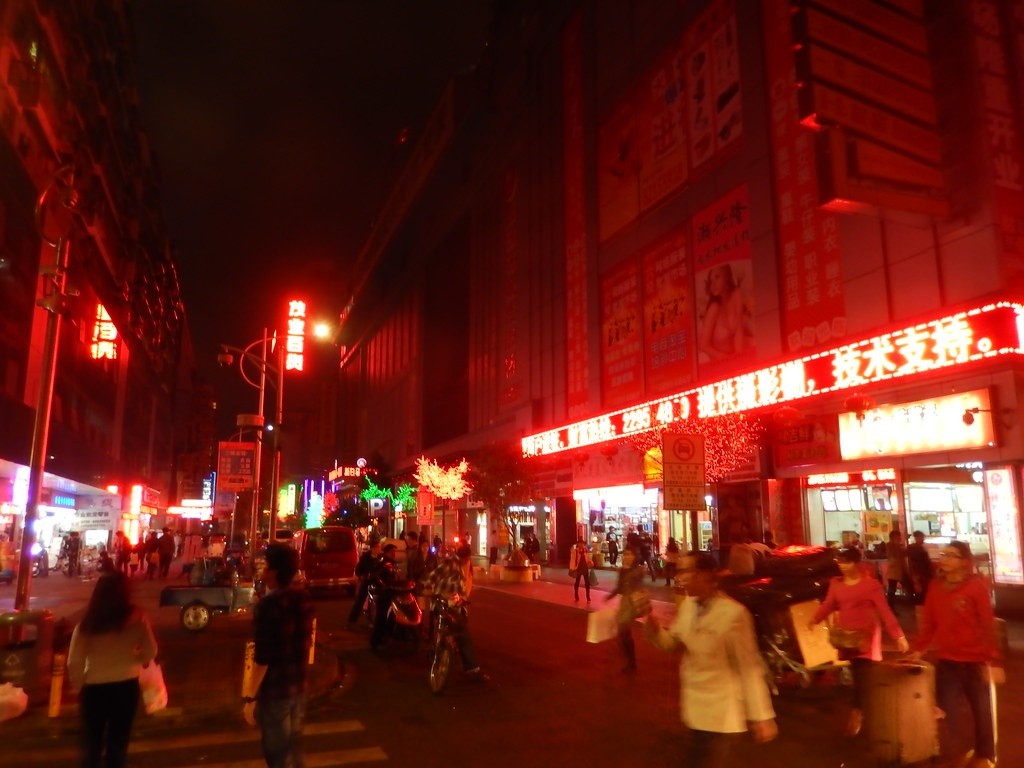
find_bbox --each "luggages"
[870,657,939,766]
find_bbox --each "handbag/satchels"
[630,589,654,620]
[137,658,169,717]
[568,568,578,578]
[590,567,599,587]
[586,604,620,644]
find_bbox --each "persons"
[907,531,932,602]
[707,539,713,551]
[606,526,620,568]
[848,534,863,548]
[66,570,157,768]
[348,527,491,684]
[243,544,315,768]
[886,531,912,600]
[489,530,498,564]
[808,546,909,735]
[646,551,778,768]
[64,527,181,578]
[908,540,1006,768]
[628,524,656,581]
[570,539,594,601]
[521,534,540,563]
[664,537,678,586]
[605,546,651,673]
[728,532,778,580]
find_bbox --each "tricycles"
[158,556,264,634]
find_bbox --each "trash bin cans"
[851,656,939,768]
[0,609,57,707]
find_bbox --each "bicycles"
[426,593,457,693]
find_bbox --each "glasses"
[937,550,962,560]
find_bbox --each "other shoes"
[575,597,580,601]
[967,757,999,768]
[467,670,492,683]
[846,717,863,737]
[587,596,591,601]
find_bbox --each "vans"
[294,525,362,597]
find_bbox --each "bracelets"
[243,697,257,703]
[900,636,905,640]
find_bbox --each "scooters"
[365,581,426,656]
